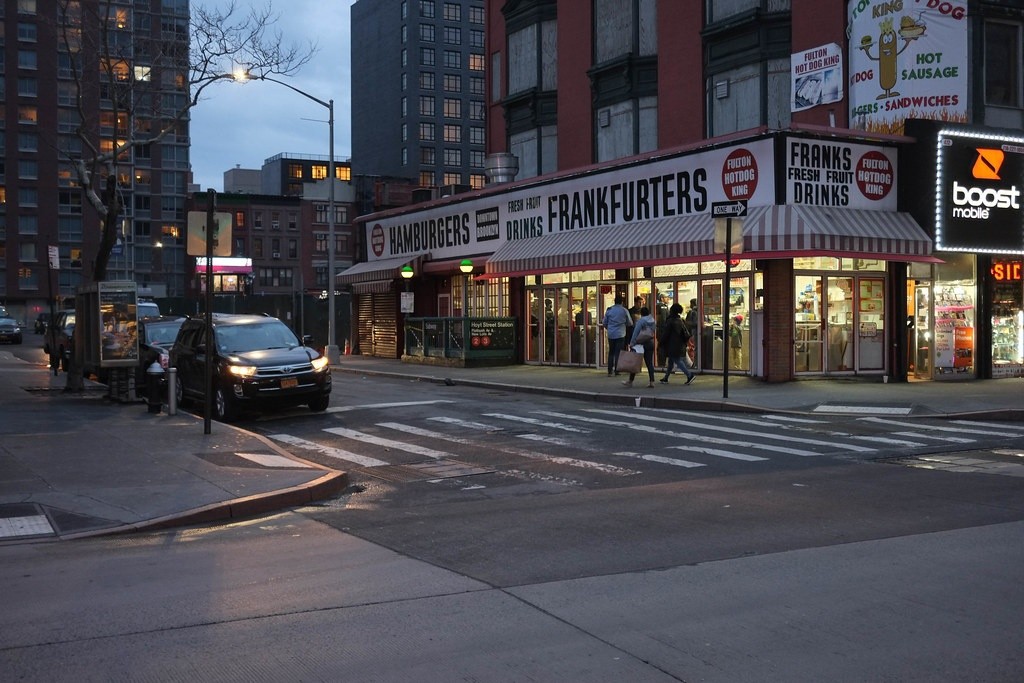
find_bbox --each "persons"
[544,286,743,389]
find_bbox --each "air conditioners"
[273,253,281,258]
[317,234,324,240]
[273,224,280,228]
[317,205,325,210]
[317,268,324,272]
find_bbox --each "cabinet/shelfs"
[814,277,884,322]
[936,287,1017,363]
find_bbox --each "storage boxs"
[572,288,582,298]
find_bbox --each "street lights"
[234,69,338,364]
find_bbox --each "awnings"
[335,253,423,295]
[472,203,946,281]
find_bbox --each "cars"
[35,312,50,334]
[138,312,191,404]
[46,309,77,373]
[0,305,23,344]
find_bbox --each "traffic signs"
[711,199,748,217]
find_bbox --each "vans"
[137,298,161,327]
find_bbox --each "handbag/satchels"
[616,347,644,373]
[636,320,654,344]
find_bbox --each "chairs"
[150,330,162,341]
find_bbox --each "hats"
[659,293,670,301]
[733,315,743,324]
[545,299,552,306]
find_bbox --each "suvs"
[170,313,333,425]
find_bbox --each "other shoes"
[685,374,696,385]
[646,381,655,388]
[659,378,669,384]
[615,372,621,375]
[608,373,612,376]
[621,380,634,387]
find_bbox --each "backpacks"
[669,317,691,343]
